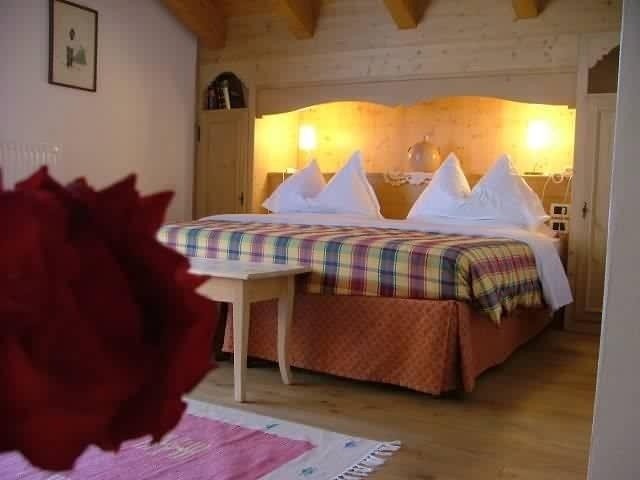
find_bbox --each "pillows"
[465,152,550,228]
[263,160,328,214]
[307,149,386,220]
[405,152,470,220]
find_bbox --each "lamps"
[297,123,319,151]
[527,116,551,147]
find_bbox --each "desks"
[187,256,306,404]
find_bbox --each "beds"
[157,170,571,397]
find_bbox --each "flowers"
[0,163,225,474]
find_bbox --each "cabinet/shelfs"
[565,91,616,336]
[192,107,252,221]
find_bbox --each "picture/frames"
[49,1,99,92]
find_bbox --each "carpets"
[1,397,399,480]
